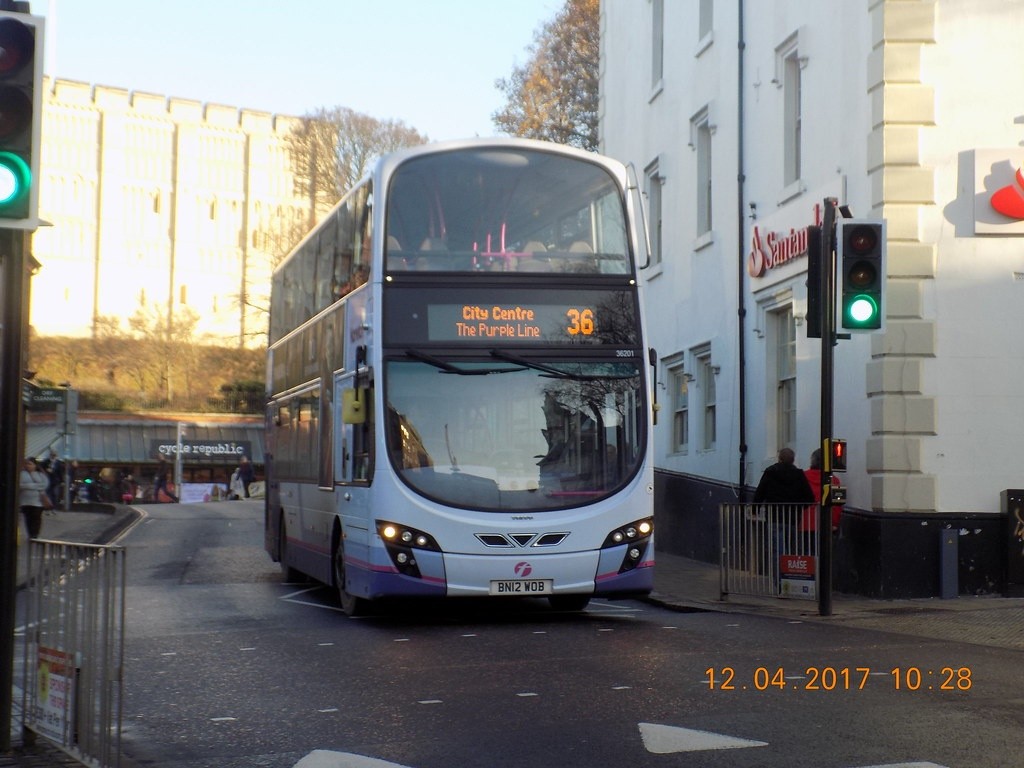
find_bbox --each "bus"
[263,137,656,616]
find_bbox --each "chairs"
[273,235,600,280]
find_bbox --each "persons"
[228,454,254,500]
[750,446,843,594]
[19,447,180,542]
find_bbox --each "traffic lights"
[842,224,881,329]
[0,10,46,233]
